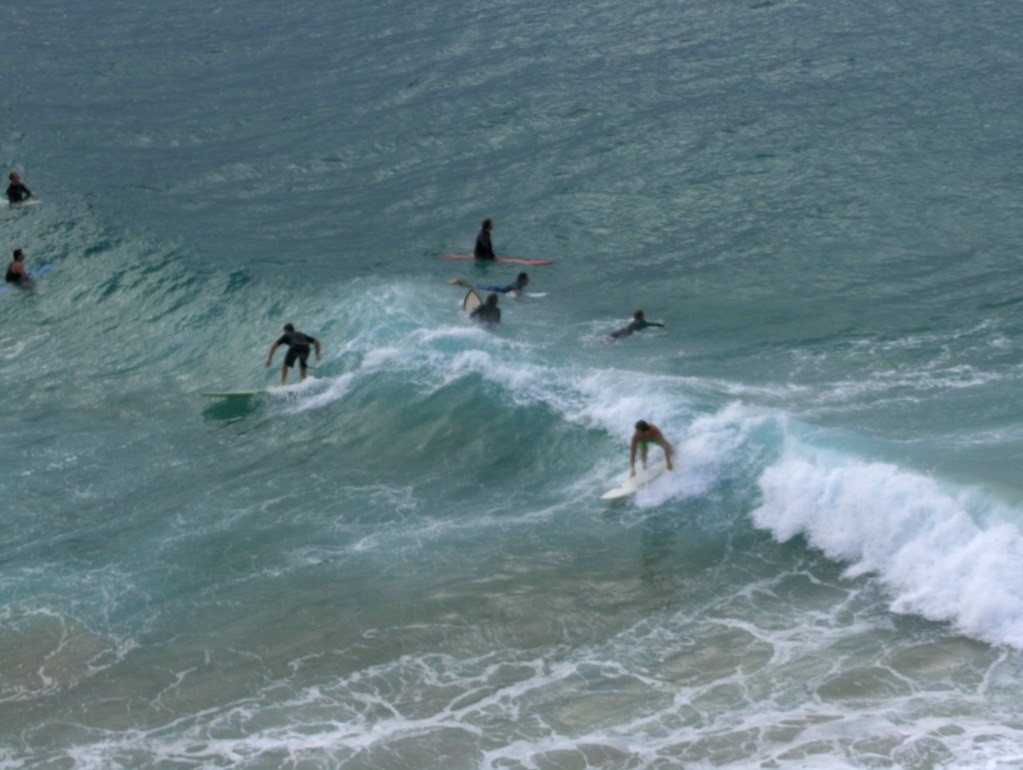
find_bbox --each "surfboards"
[198,386,272,399]
[0,256,65,298]
[463,290,482,317]
[599,453,673,503]
[0,196,43,209]
[470,286,553,301]
[435,251,550,269]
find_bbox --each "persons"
[611,310,669,338]
[469,293,500,326]
[630,420,673,476]
[448,272,528,297]
[266,324,321,385]
[448,219,552,265]
[7,172,32,204]
[5,248,27,282]
